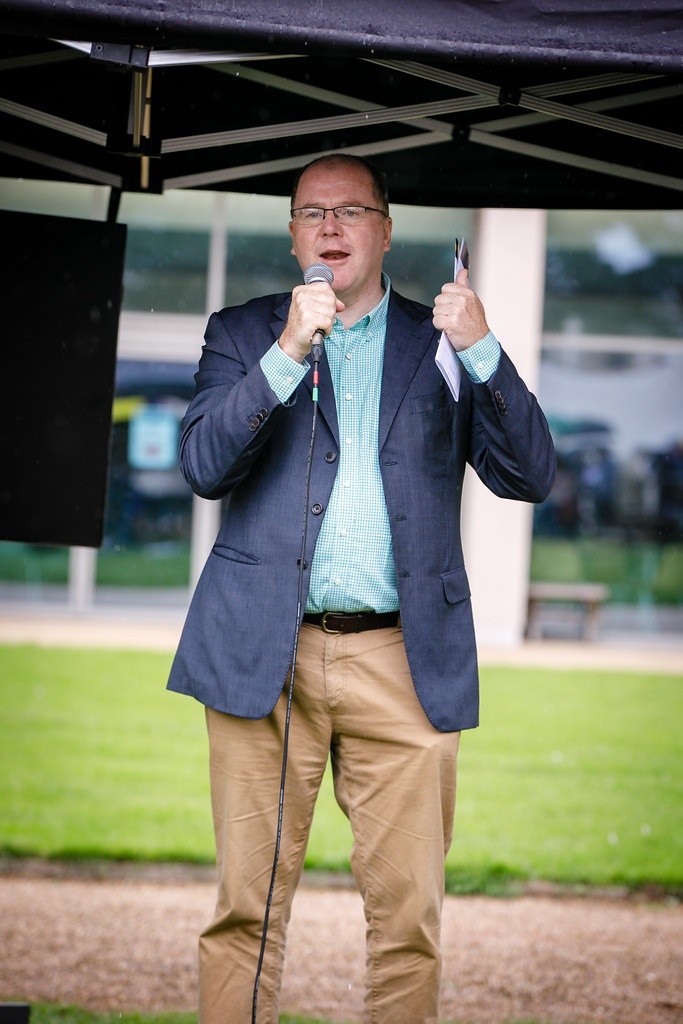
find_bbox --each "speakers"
[0,209,128,546]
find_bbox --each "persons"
[164,153,559,1024]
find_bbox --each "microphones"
[303,262,334,363]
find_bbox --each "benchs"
[526,582,606,642]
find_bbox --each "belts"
[300,611,405,636]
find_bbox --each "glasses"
[290,204,388,225]
